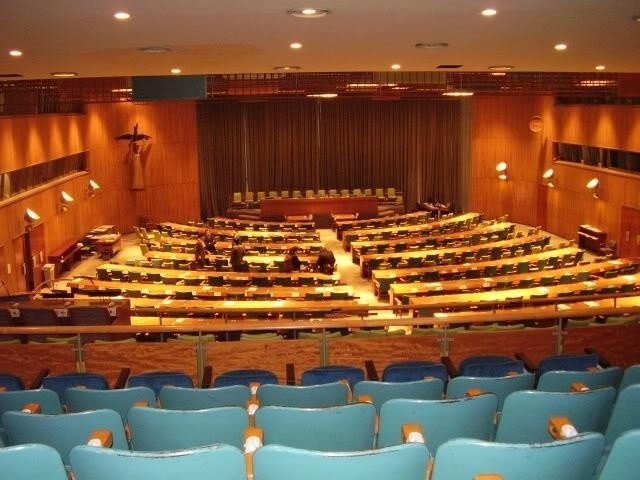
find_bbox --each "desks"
[363,231,549,279]
[96,263,340,282]
[389,258,635,314]
[159,222,319,238]
[206,216,315,228]
[73,296,370,311]
[408,273,640,332]
[353,221,519,265]
[261,196,379,215]
[66,278,354,297]
[285,215,308,222]
[145,250,318,265]
[432,297,640,329]
[169,238,324,247]
[344,212,482,252]
[577,224,606,249]
[2,297,129,332]
[131,317,375,336]
[372,244,582,299]
[332,213,356,220]
[335,207,428,240]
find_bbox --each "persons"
[230,237,246,271]
[195,233,206,266]
[204,230,215,252]
[289,246,302,273]
[231,227,242,245]
[309,248,335,274]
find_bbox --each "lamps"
[273,65,300,72]
[51,72,78,78]
[489,66,514,70]
[287,5,330,20]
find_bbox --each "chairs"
[125,259,135,267]
[253,276,269,285]
[299,221,317,230]
[318,277,333,284]
[516,347,607,372]
[112,270,123,281]
[268,222,279,231]
[203,257,212,269]
[229,220,238,227]
[139,261,150,266]
[419,214,425,223]
[379,373,497,445]
[511,244,519,254]
[30,366,108,388]
[285,362,365,384]
[369,331,405,337]
[528,226,535,235]
[252,236,265,243]
[518,279,534,287]
[456,220,463,227]
[364,188,371,195]
[593,256,604,263]
[489,218,497,224]
[375,188,386,202]
[359,223,367,227]
[239,333,283,342]
[44,338,81,343]
[446,271,461,279]
[579,290,596,295]
[146,272,163,284]
[506,233,515,240]
[251,424,427,480]
[506,296,523,308]
[426,254,438,261]
[160,231,168,239]
[140,227,153,241]
[567,239,573,248]
[578,271,591,280]
[425,271,438,281]
[181,277,201,285]
[249,262,266,271]
[440,353,525,375]
[232,192,242,204]
[273,276,292,283]
[429,239,437,249]
[621,265,635,274]
[616,364,640,389]
[466,218,471,225]
[443,237,453,247]
[472,235,480,242]
[125,403,249,454]
[151,245,158,252]
[257,191,265,201]
[206,217,216,226]
[64,386,157,411]
[259,378,353,403]
[491,235,499,242]
[368,258,379,267]
[607,313,639,325]
[387,256,399,264]
[352,188,361,195]
[151,259,171,268]
[227,293,245,300]
[386,187,398,201]
[253,293,274,300]
[604,271,617,278]
[364,357,447,382]
[398,230,409,236]
[215,259,229,271]
[409,217,416,224]
[366,233,375,240]
[340,189,350,196]
[493,247,500,258]
[535,260,547,269]
[176,334,214,343]
[606,253,613,261]
[305,293,323,299]
[318,189,324,195]
[245,191,254,204]
[238,235,249,241]
[2,404,128,449]
[477,248,489,256]
[518,262,529,271]
[0,340,22,346]
[466,268,480,277]
[300,333,342,340]
[253,245,269,254]
[157,382,257,406]
[342,223,354,231]
[306,189,314,198]
[447,370,536,398]
[541,277,553,285]
[395,244,407,251]
[621,283,636,293]
[0,432,66,479]
[152,229,162,242]
[131,225,142,238]
[69,427,252,479]
[292,190,300,197]
[470,325,494,332]
[421,229,428,234]
[564,254,571,265]
[397,218,405,225]
[559,274,572,283]
[381,231,391,239]
[557,291,574,300]
[253,222,265,230]
[311,246,323,254]
[155,223,162,230]
[0,390,63,412]
[385,219,393,226]
[208,275,225,284]
[474,216,480,225]
[601,288,617,293]
[576,252,585,261]
[139,243,148,255]
[298,277,315,285]
[287,235,303,242]
[360,246,374,254]
[496,281,512,290]
[280,190,289,198]
[496,324,525,331]
[613,382,640,435]
[281,224,295,232]
[376,244,389,252]
[484,265,496,277]
[219,220,227,226]
[328,189,336,195]
[500,214,506,223]
[548,256,558,266]
[355,330,384,338]
[444,252,455,258]
[432,327,461,333]
[28,340,68,346]
[461,251,473,257]
[517,230,522,238]
[299,260,310,268]
[410,328,434,336]
[267,260,283,271]
[96,267,108,279]
[524,242,530,252]
[530,294,549,306]
[566,318,596,328]
[502,264,513,274]
[408,256,422,264]
[219,233,227,240]
[201,366,277,388]
[599,240,615,254]
[270,235,285,242]
[184,242,198,250]
[128,290,142,296]
[331,292,349,300]
[543,245,551,251]
[175,292,193,299]
[431,411,607,478]
[241,222,250,227]
[269,191,277,197]
[230,278,249,285]
[107,287,123,294]
[173,259,193,269]
[598,430,637,479]
[535,367,622,390]
[126,271,142,281]
[113,366,195,391]
[559,241,566,249]
[353,376,444,400]
[498,380,617,440]
[253,397,378,453]
